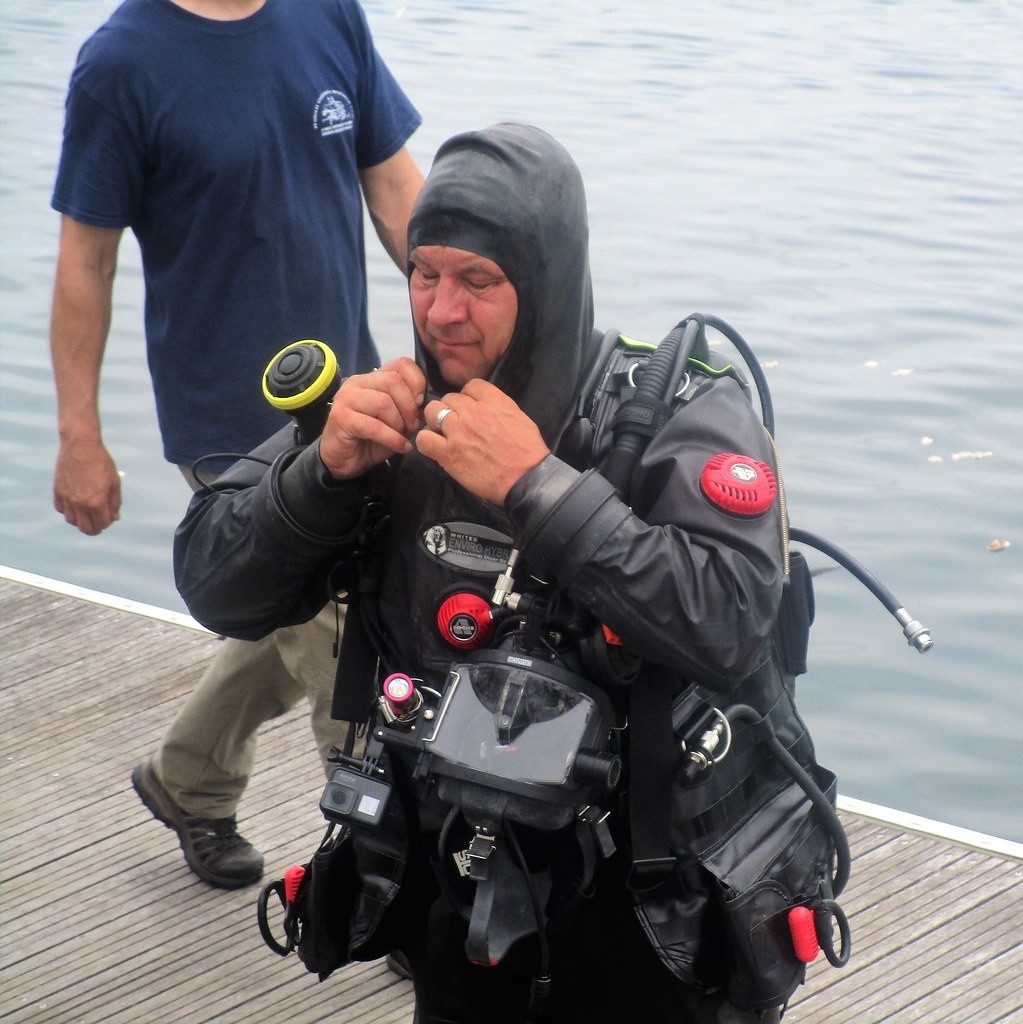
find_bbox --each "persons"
[50,0,425,887]
[172,124,811,1024]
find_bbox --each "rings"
[436,408,454,426]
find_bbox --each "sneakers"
[131,755,264,889]
